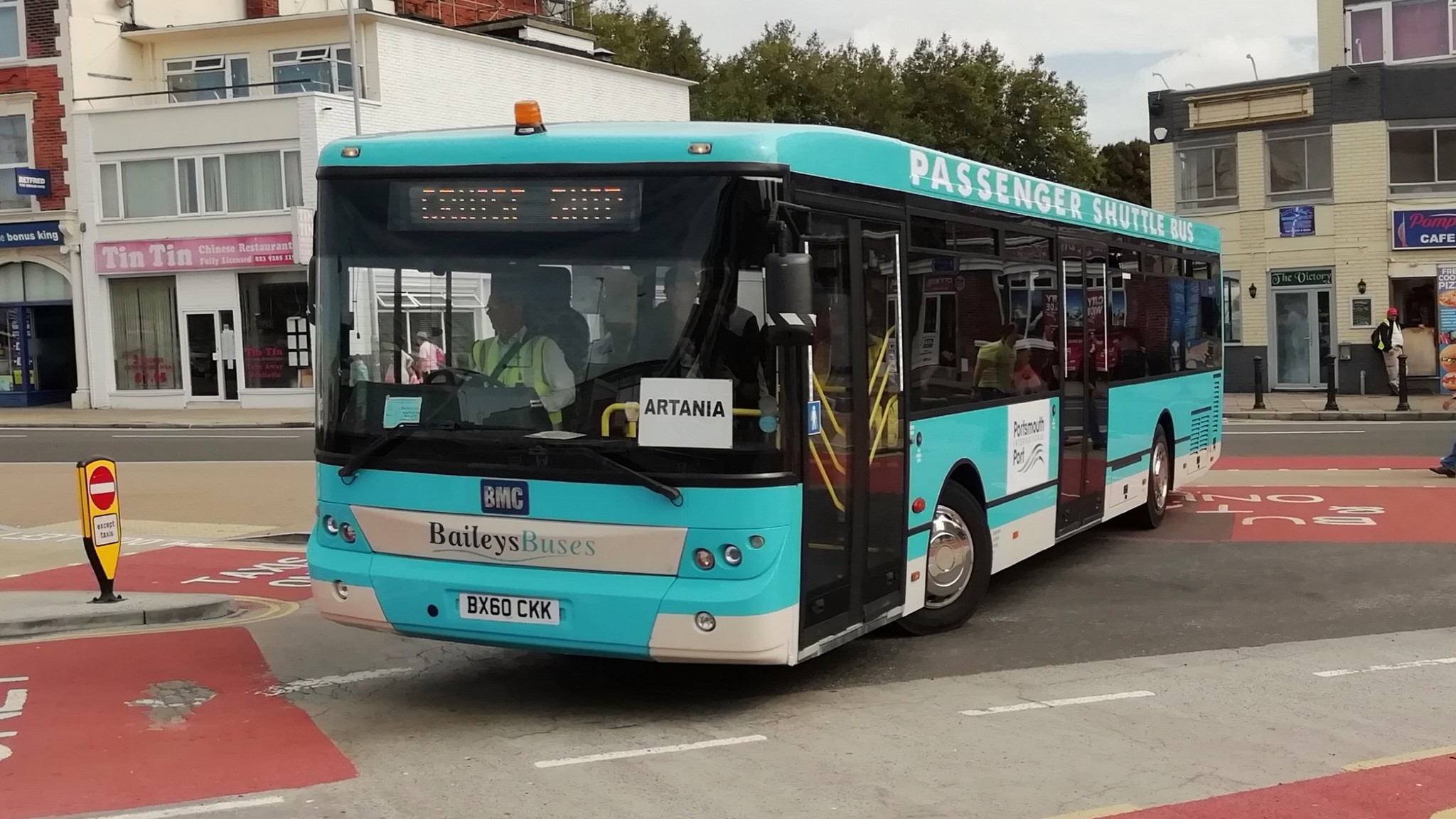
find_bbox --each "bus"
[306,100,1226,671]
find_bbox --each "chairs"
[525,265,590,379]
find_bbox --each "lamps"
[1248,283,1257,298]
[1347,72,1364,81]
[1357,279,1366,294]
[1149,98,1163,116]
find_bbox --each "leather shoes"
[1430,466,1456,477]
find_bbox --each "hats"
[417,331,431,341]
[1387,308,1398,315]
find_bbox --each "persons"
[1370,308,1409,396]
[384,332,446,385]
[468,288,577,432]
[1429,393,1456,478]
[970,322,1106,450]
[628,265,770,411]
[998,333,1042,399]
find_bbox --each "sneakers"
[1390,382,1399,395]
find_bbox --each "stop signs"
[89,466,116,510]
